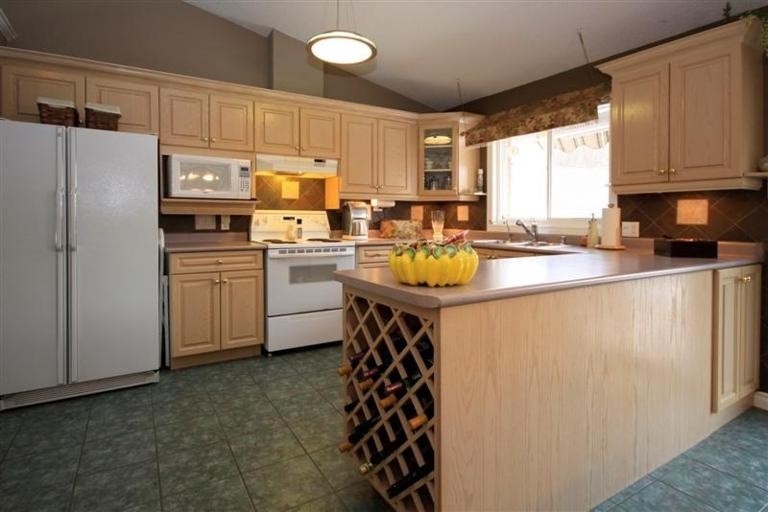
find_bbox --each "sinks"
[512,242,568,249]
[473,240,522,246]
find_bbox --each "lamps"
[306,3,378,67]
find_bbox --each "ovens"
[264,249,356,317]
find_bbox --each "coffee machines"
[341,200,369,240]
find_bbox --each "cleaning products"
[587,213,598,248]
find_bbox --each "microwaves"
[168,153,252,199]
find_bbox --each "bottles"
[476,168,482,192]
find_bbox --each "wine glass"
[431,209,447,240]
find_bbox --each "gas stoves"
[259,231,355,247]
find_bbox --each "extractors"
[255,151,340,188]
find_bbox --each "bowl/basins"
[423,157,451,170]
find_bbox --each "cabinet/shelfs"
[338,111,419,201]
[418,121,481,202]
[167,249,266,372]
[339,273,716,511]
[608,46,763,196]
[0,55,161,140]
[355,245,394,273]
[158,79,342,167]
[710,264,762,415]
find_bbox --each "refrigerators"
[3,114,162,412]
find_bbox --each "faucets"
[516,220,538,242]
